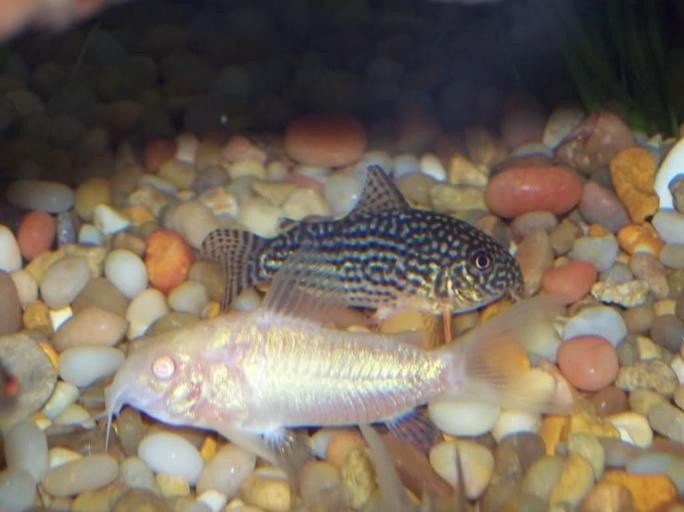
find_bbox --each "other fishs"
[78,240,585,512]
[0,333,58,436]
[192,165,525,316]
